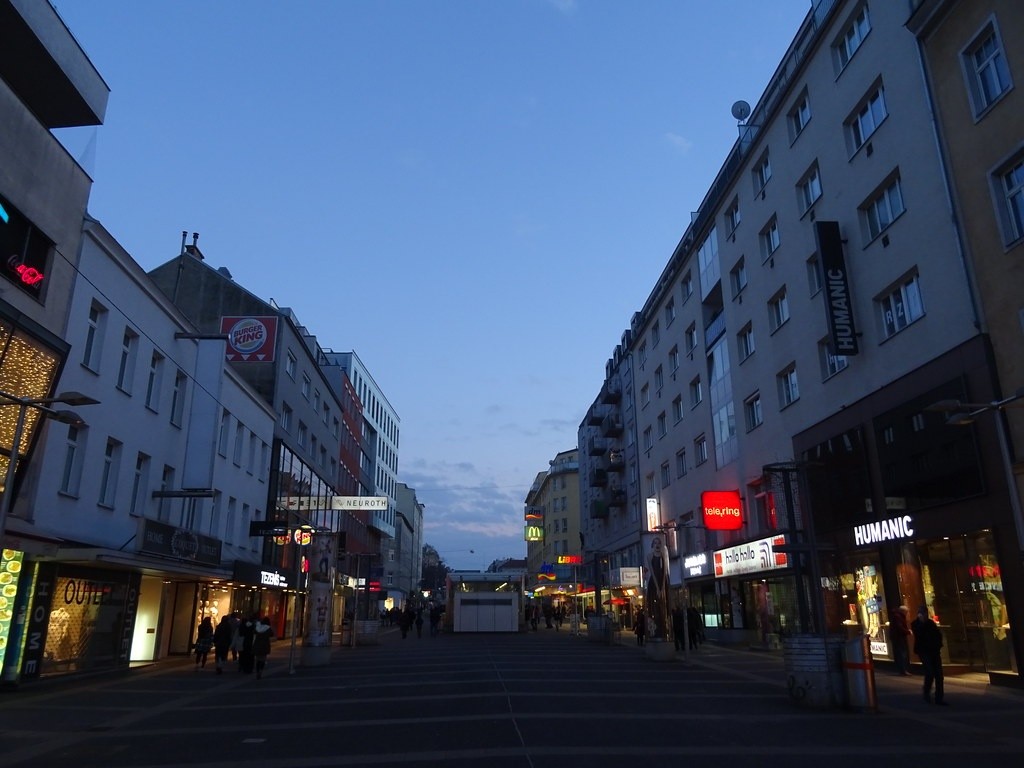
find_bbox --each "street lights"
[651,525,692,662]
[561,562,580,638]
[352,553,376,650]
[285,525,332,677]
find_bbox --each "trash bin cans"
[826,633,878,720]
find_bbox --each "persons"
[647,537,669,599]
[211,611,274,678]
[911,605,944,702]
[890,605,914,676]
[193,617,213,667]
[380,601,705,651]
